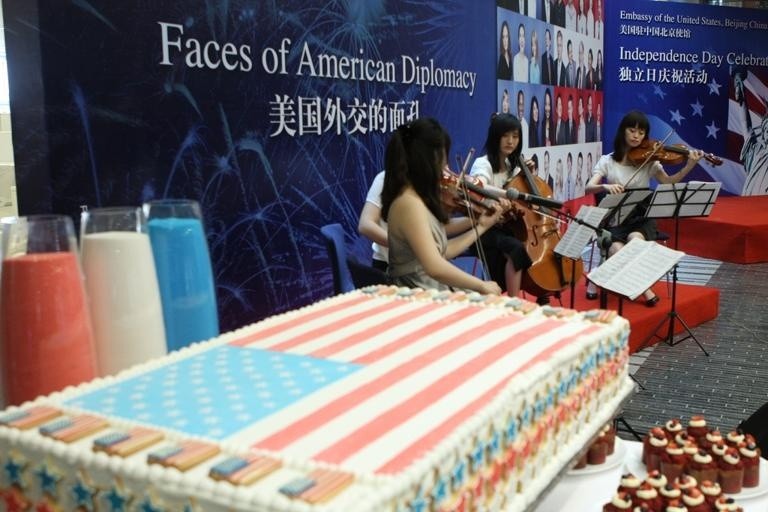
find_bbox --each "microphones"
[506,187,564,209]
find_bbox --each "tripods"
[636,214,710,358]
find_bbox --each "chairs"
[321,224,384,293]
[600,178,672,300]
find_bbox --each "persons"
[466,112,552,306]
[498,0,606,207]
[355,129,502,287]
[378,114,512,297]
[579,111,707,307]
[734,75,767,197]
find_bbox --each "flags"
[606,54,768,197]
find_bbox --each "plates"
[566,432,626,476]
[623,451,768,501]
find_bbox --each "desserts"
[573,415,761,511]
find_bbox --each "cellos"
[490,112,583,296]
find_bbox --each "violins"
[437,166,525,224]
[627,139,723,168]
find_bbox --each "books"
[585,237,685,302]
[552,201,610,260]
[648,180,722,217]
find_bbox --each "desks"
[532,440,768,512]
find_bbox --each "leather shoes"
[646,294,661,306]
[585,290,599,299]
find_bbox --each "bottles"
[141,199,220,352]
[78,205,169,378]
[0,213,100,411]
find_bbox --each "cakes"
[0,283,637,511]
